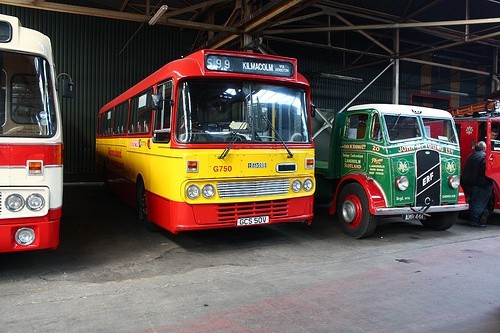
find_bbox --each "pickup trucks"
[316,101,470,238]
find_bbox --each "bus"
[97,49,316,237]
[0,14,75,253]
[444,101,500,213]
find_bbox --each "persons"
[483,129,497,150]
[462,141,496,227]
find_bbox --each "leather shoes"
[468,221,486,227]
